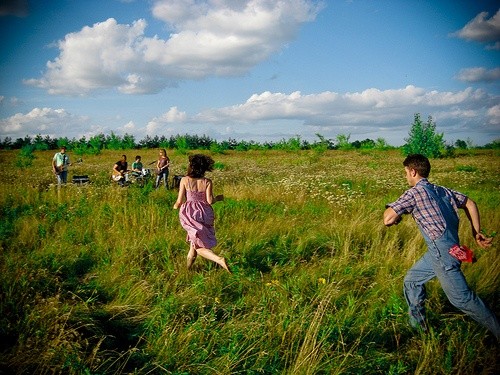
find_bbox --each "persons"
[383,154,500,340]
[155,149,170,191]
[52,146,72,184]
[112,155,144,187]
[173,154,232,274]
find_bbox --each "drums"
[141,168,152,180]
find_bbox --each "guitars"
[112,166,138,182]
[155,162,172,176]
[53,158,83,175]
